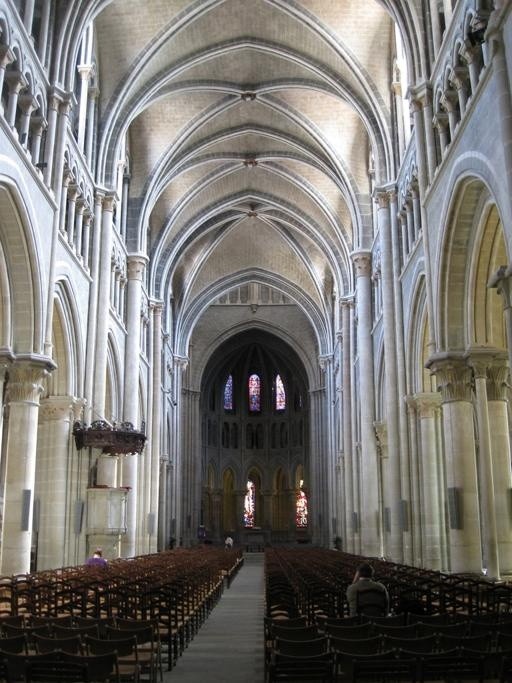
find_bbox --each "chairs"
[264,542,510,681]
[1,558,211,683]
[135,541,245,605]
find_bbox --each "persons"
[223,533,234,550]
[346,562,391,616]
[85,548,111,588]
[333,536,343,551]
